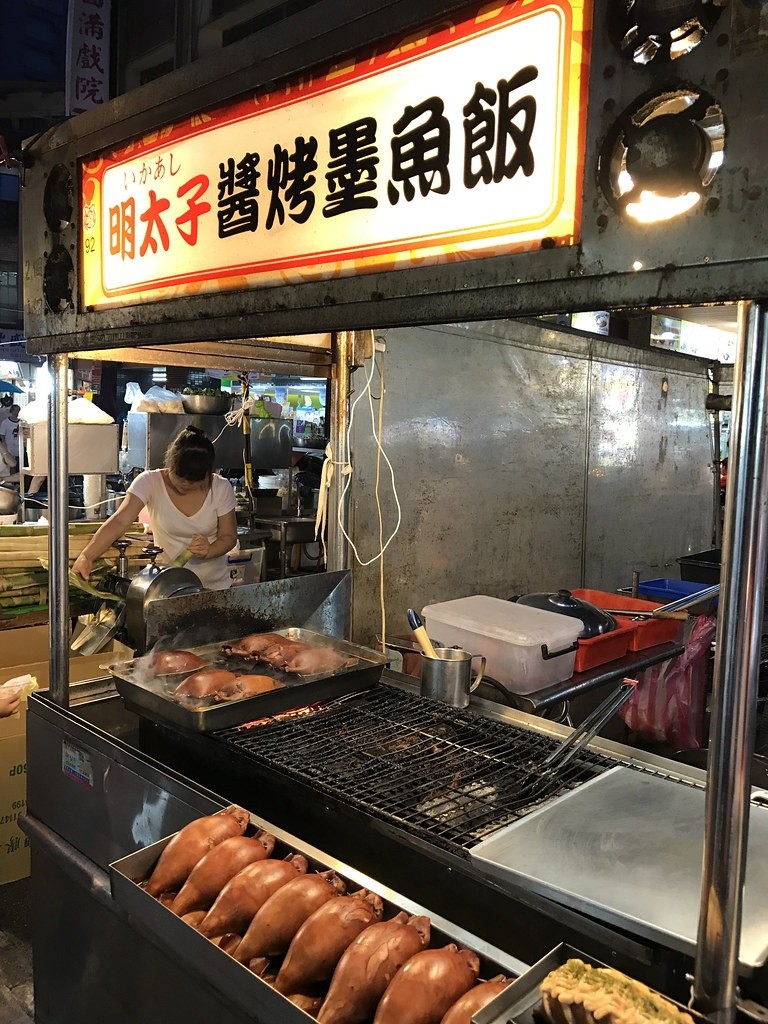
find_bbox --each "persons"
[0,394,13,425]
[0,404,30,475]
[72,425,237,589]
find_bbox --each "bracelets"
[1,451,10,457]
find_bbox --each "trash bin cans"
[676,547,733,585]
[618,578,721,614]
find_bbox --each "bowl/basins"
[180,393,232,414]
[254,489,278,497]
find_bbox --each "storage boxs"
[420,594,582,694]
[638,578,720,607]
[0,622,136,886]
[574,615,639,673]
[569,588,687,651]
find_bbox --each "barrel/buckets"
[672,747,768,790]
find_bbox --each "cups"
[421,647,486,709]
[298,438,304,447]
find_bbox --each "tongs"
[520,678,640,798]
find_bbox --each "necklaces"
[9,415,19,422]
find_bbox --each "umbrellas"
[0,379,26,393]
